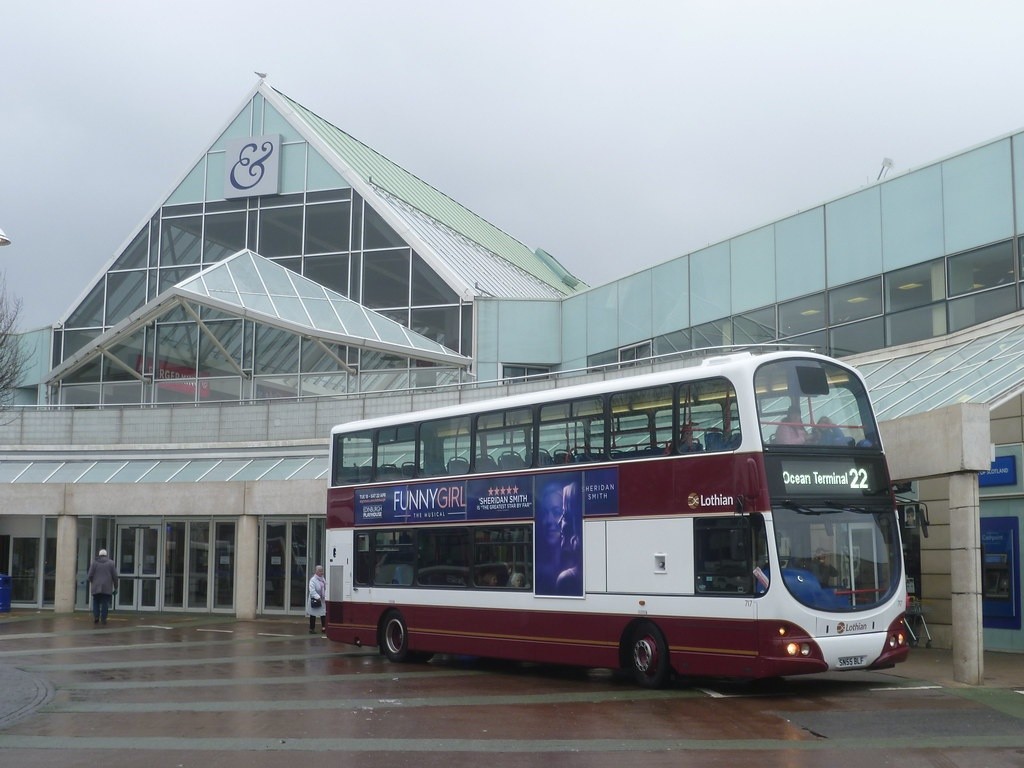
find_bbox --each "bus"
[322,349,932,687]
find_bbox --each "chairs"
[704,427,723,451]
[475,453,498,473]
[731,428,741,449]
[380,463,401,482]
[644,446,662,456]
[359,466,379,482]
[603,450,623,461]
[402,461,424,480]
[566,447,588,462]
[529,448,554,466]
[770,434,776,450]
[554,449,575,463]
[498,455,517,470]
[448,456,470,474]
[501,451,525,470]
[589,447,603,461]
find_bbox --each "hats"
[680,427,691,433]
[99,549,107,555]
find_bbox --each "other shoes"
[95,617,99,623]
[309,629,317,634]
[101,621,107,625]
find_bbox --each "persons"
[774,405,845,445]
[906,507,916,526]
[441,559,524,587]
[87,550,117,624]
[307,565,326,633]
[809,547,839,587]
[537,480,580,596]
[661,425,693,455]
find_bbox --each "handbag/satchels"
[311,598,321,608]
